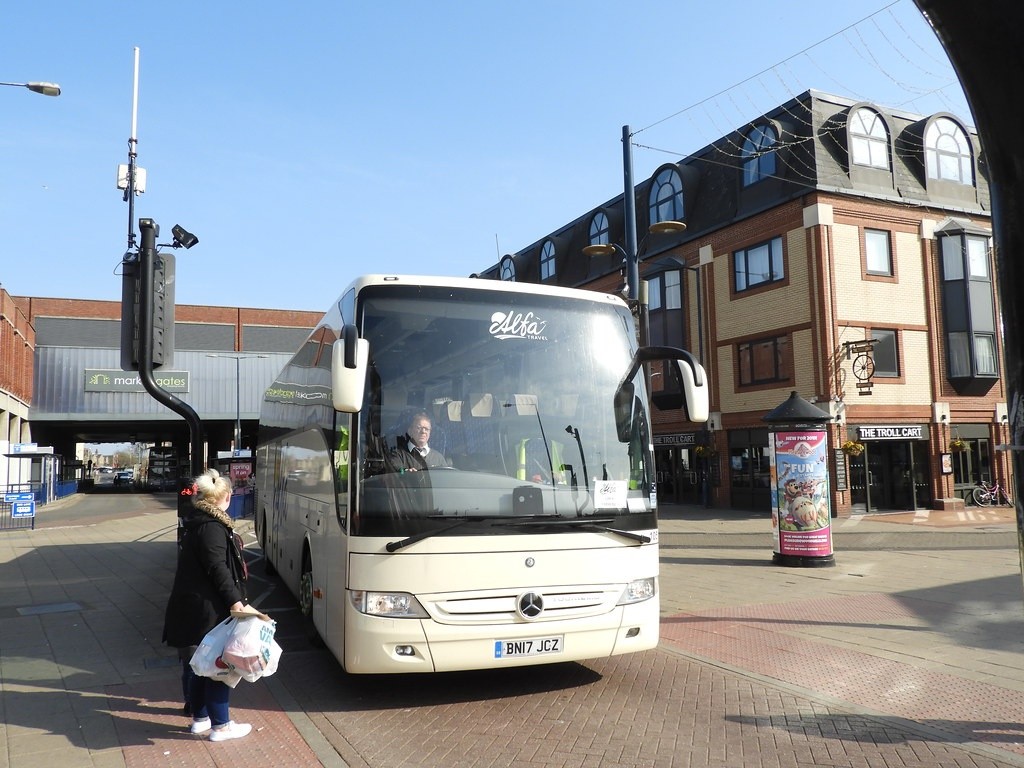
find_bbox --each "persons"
[162,470,252,742]
[383,410,447,474]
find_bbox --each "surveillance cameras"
[172,224,199,250]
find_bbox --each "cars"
[113,471,132,484]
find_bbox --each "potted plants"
[695,442,711,458]
[949,441,968,452]
[841,441,864,456]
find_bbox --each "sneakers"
[191,719,211,733]
[209,722,252,741]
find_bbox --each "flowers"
[181,483,199,496]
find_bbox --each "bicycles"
[972,479,1015,508]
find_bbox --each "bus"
[254,275,710,677]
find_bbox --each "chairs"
[498,393,538,476]
[383,407,446,456]
[432,397,462,454]
[460,393,501,456]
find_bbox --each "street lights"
[581,124,688,341]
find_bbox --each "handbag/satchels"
[188,615,283,689]
